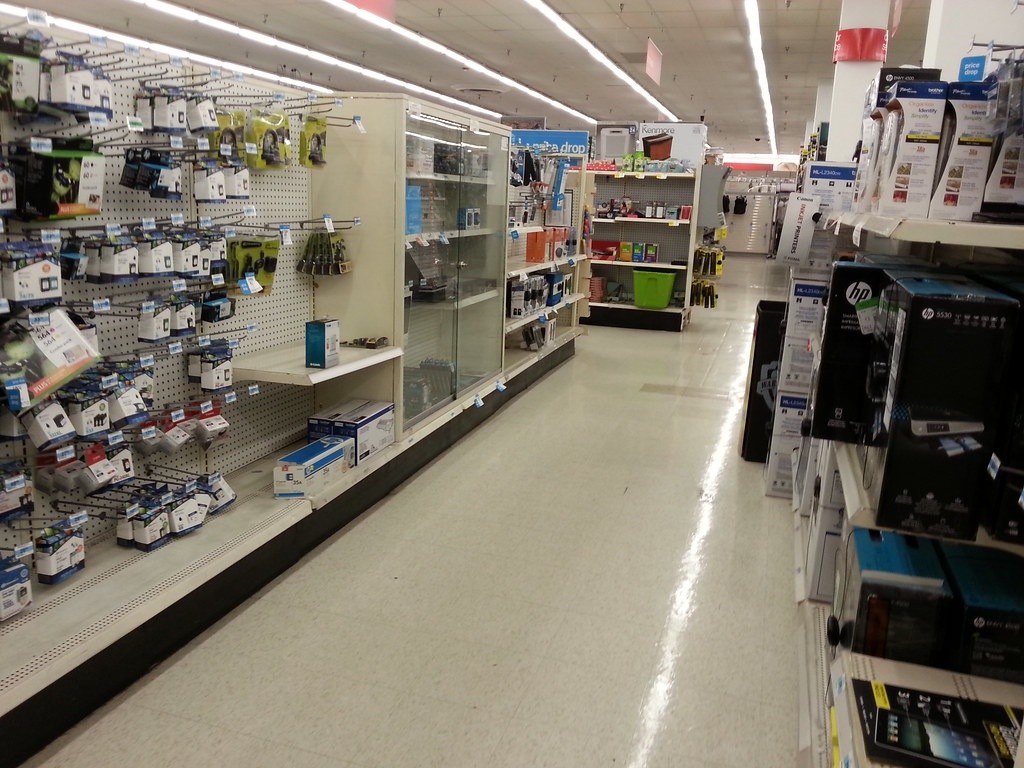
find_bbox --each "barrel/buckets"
[632,267,676,310]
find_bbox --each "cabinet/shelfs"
[189,58,403,549]
[0,10,313,768]
[568,161,732,333]
[504,130,585,401]
[317,92,512,488]
[791,205,1024,768]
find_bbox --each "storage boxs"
[408,137,488,177]
[645,202,693,221]
[306,318,341,369]
[504,271,572,320]
[457,208,480,231]
[691,228,727,307]
[742,40,1024,768]
[413,276,495,304]
[619,241,659,265]
[521,318,557,351]
[598,198,632,218]
[0,56,248,622]
[526,227,576,263]
[272,399,395,500]
